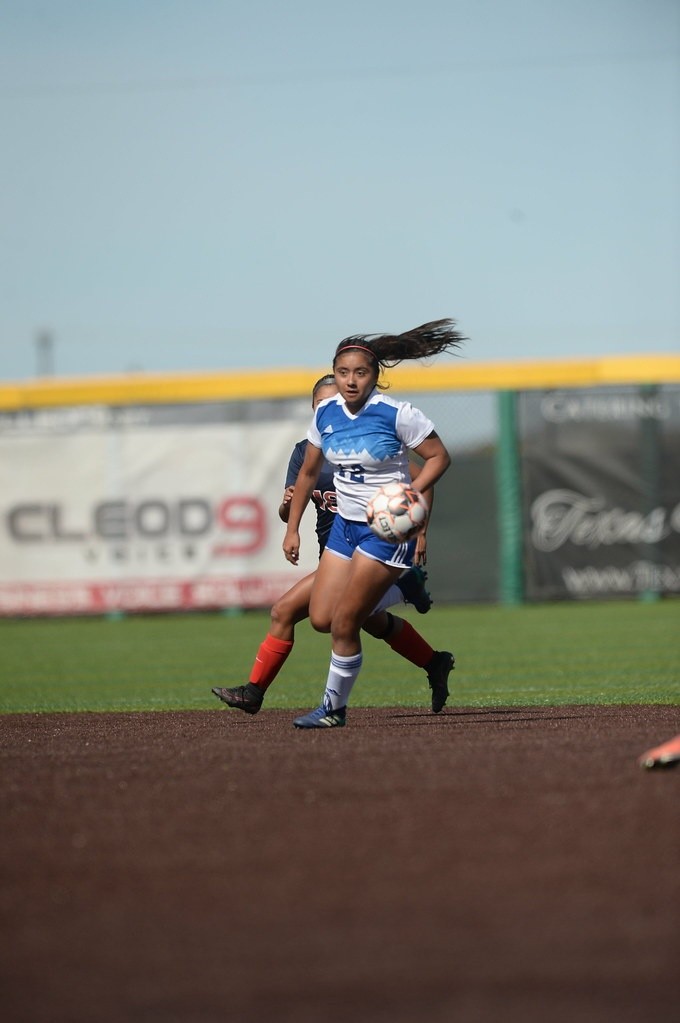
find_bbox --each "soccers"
[366,481,430,544]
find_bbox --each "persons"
[209,320,470,731]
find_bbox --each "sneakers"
[424,651,455,713]
[395,565,433,614]
[212,685,264,716]
[293,704,346,728]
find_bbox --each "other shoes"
[638,734,680,769]
